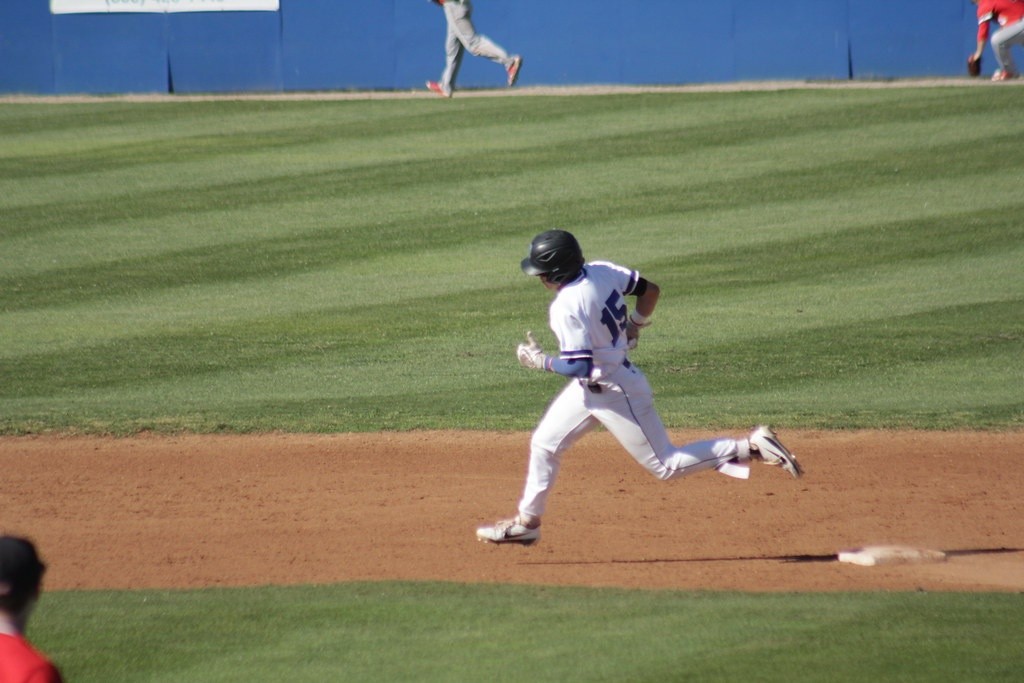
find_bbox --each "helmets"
[521,230,585,285]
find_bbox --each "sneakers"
[476,516,541,546]
[749,426,801,480]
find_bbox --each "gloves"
[517,331,547,370]
[625,321,640,350]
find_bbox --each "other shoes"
[426,82,452,98]
[504,55,522,87]
[991,66,1019,81]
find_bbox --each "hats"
[0,535,46,606]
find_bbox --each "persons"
[968,0,1024,82]
[426,0,522,97]
[0,536,63,683]
[476,230,806,548]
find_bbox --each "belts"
[579,358,631,393]
